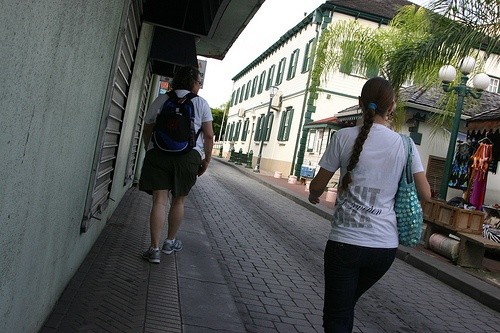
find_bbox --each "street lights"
[251,84,279,173]
[437,56,490,202]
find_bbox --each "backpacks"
[153,90,204,152]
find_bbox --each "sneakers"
[140,247,161,263]
[162,239,184,254]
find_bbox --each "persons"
[308,77,431,333]
[138,65,213,264]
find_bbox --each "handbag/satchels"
[395,134,423,248]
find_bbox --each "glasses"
[194,79,203,87]
